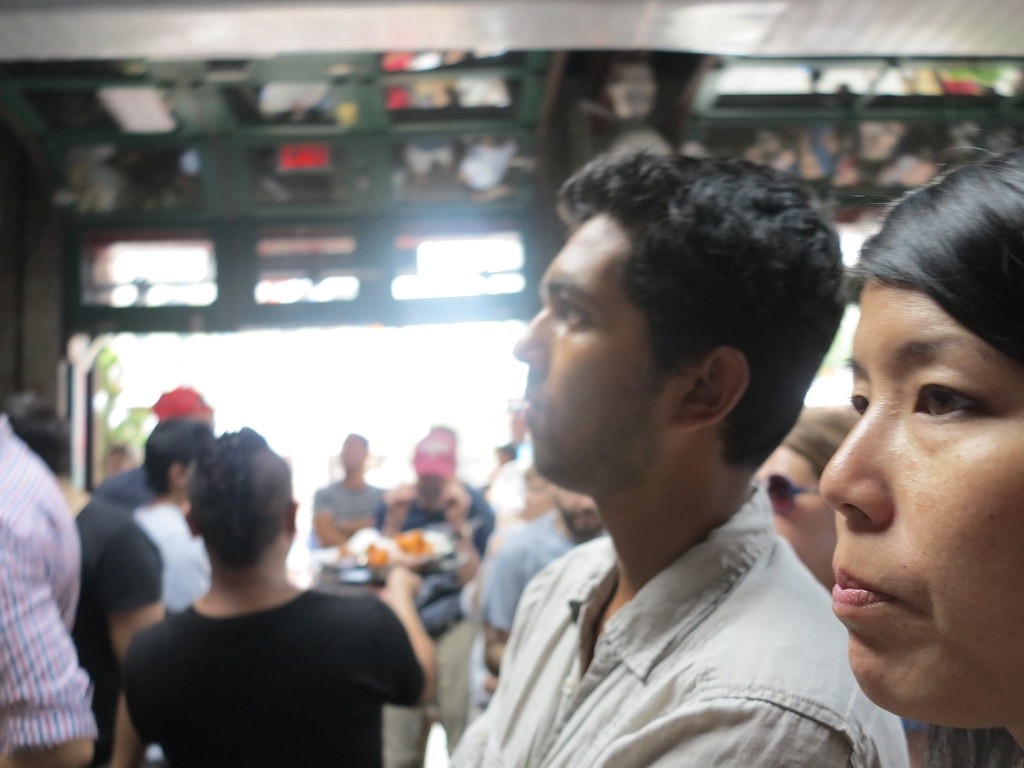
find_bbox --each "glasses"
[768,475,824,516]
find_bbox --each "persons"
[0,149,1024,768]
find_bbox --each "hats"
[413,432,455,476]
[152,387,214,424]
